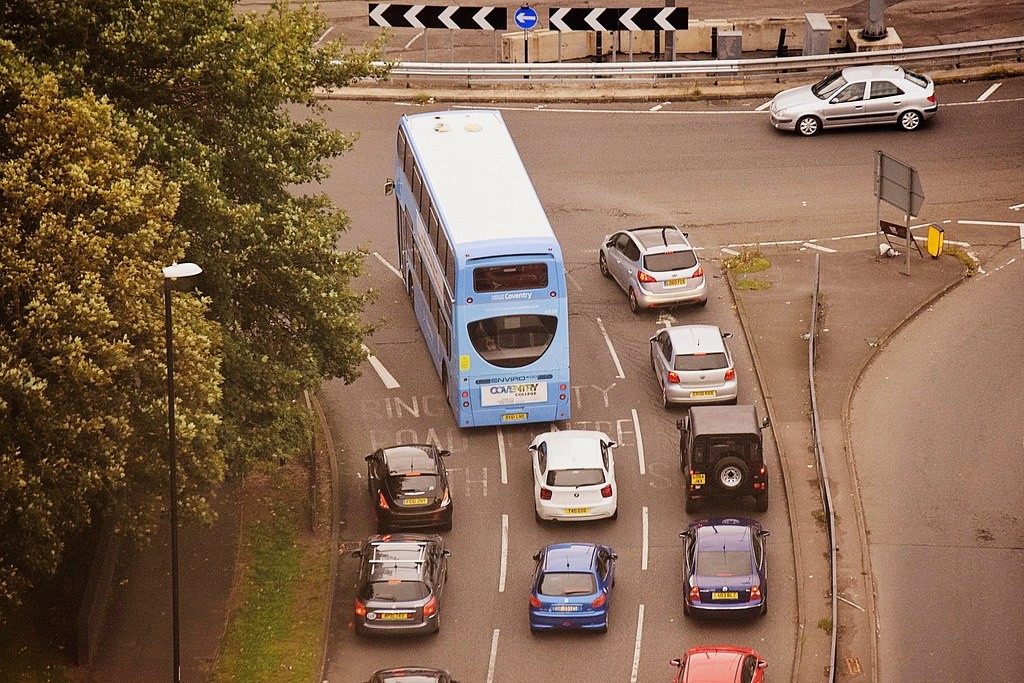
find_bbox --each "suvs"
[674,404,769,515]
[598,223,711,312]
[526,430,620,523]
[352,533,450,637]
[364,444,454,532]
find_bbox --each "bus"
[381,108,574,428]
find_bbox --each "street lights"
[159,261,208,679]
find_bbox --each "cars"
[681,515,770,620]
[367,667,459,682]
[668,647,770,683]
[528,542,618,632]
[649,322,738,409]
[767,62,938,135]
